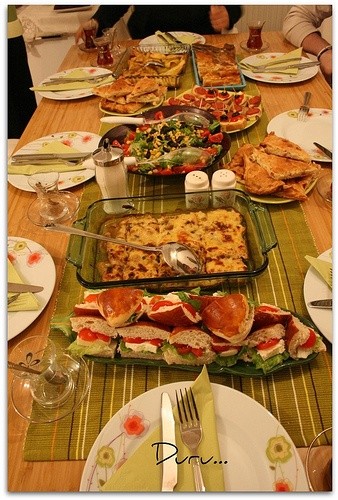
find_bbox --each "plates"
[78,381,309,492]
[26,191,79,230]
[239,52,320,84]
[50,292,323,377]
[191,47,246,91]
[305,427,331,491]
[7,236,56,343]
[240,39,269,54]
[10,348,92,424]
[139,31,206,54]
[38,67,112,101]
[168,88,262,134]
[90,56,118,69]
[79,41,99,53]
[303,247,332,343]
[7,131,102,193]
[99,91,164,117]
[235,162,319,204]
[316,172,332,209]
[112,44,191,90]
[266,108,333,162]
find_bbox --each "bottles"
[93,138,135,215]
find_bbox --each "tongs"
[192,43,224,52]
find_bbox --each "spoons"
[97,61,164,89]
[100,112,209,127]
[84,147,212,169]
[45,223,202,276]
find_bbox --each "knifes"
[12,153,89,160]
[308,298,333,308]
[7,283,43,293]
[313,142,332,160]
[162,392,178,492]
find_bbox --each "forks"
[31,153,92,165]
[172,387,205,493]
[248,58,301,68]
[298,92,312,119]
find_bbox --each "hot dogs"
[73,290,98,316]
[202,294,254,344]
[144,291,200,327]
[68,317,115,358]
[209,334,241,365]
[286,316,327,360]
[241,325,286,370]
[251,303,292,324]
[116,324,168,360]
[165,329,214,366]
[96,287,147,329]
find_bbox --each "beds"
[6,30,331,491]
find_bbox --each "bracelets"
[315,43,331,62]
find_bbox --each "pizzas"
[220,133,320,203]
[90,74,165,113]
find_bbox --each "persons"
[280,5,333,78]
[72,5,245,49]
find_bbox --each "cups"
[93,37,113,66]
[10,336,73,407]
[246,19,265,49]
[82,21,99,48]
[103,28,119,52]
[28,171,67,220]
